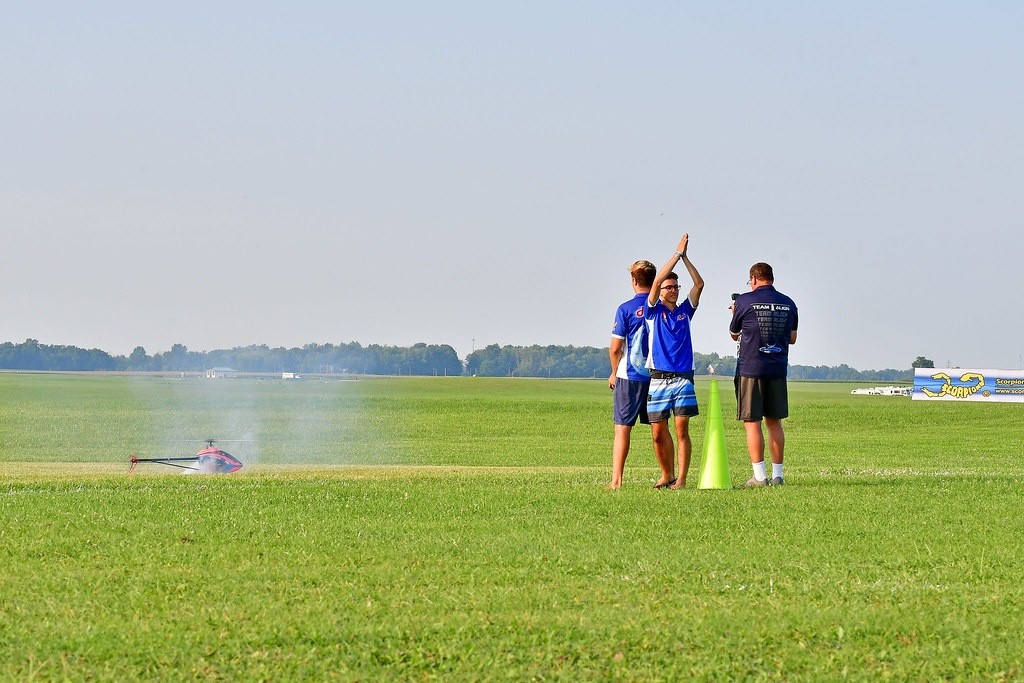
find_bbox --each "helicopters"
[126,439,256,477]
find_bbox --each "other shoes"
[738,478,768,488]
[653,478,676,491]
[767,477,782,485]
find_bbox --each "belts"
[651,372,689,379]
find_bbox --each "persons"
[644,232,704,491]
[727,262,799,487]
[607,261,675,491]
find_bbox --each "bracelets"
[675,250,683,257]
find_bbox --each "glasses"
[661,284,681,291]
[746,279,751,287]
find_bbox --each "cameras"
[729,293,740,309]
